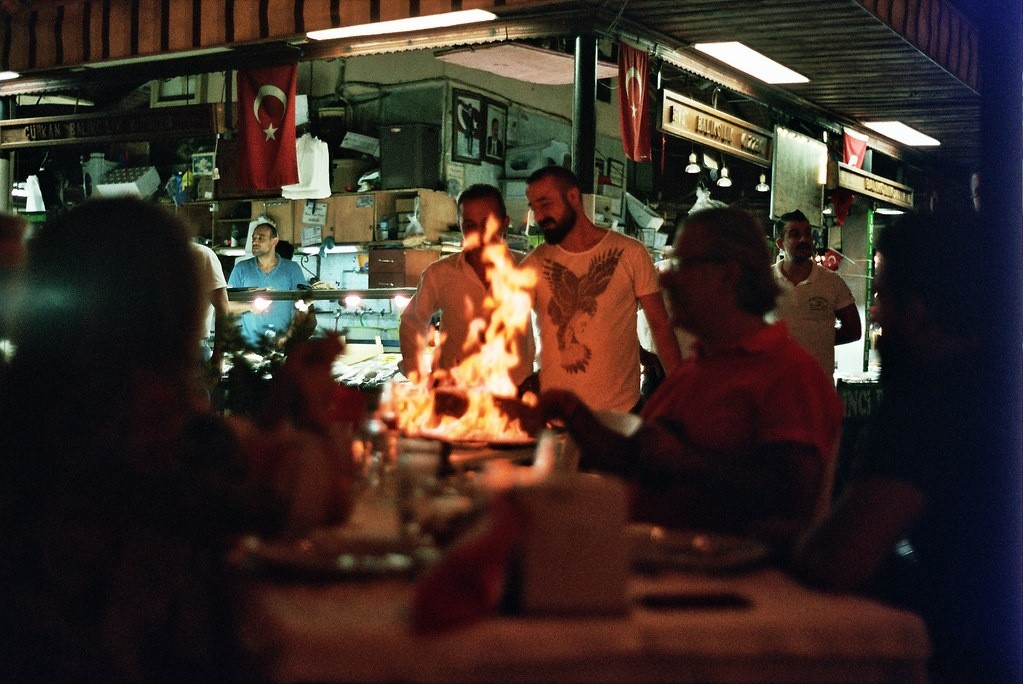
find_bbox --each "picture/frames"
[452,87,484,165]
[484,97,508,166]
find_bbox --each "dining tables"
[229,440,923,684]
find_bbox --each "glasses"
[662,251,738,275]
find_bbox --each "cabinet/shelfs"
[368,247,440,289]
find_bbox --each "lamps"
[755,167,770,192]
[685,144,701,173]
[716,153,732,187]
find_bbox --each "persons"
[486,118,504,158]
[465,104,474,156]
[0,199,356,684]
[399,166,1023,684]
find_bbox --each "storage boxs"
[251,189,458,246]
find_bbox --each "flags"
[618,40,655,165]
[235,62,300,191]
[834,127,868,224]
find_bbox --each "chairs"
[786,473,922,592]
